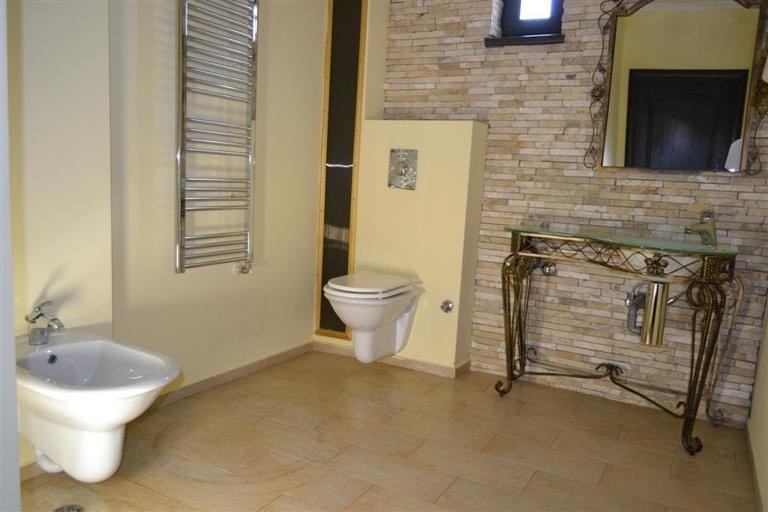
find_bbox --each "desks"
[495,226,737,458]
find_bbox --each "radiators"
[173,0,259,275]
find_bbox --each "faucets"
[684,209,718,245]
[24,299,65,346]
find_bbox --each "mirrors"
[583,0,768,176]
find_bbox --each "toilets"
[323,269,425,363]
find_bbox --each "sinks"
[16,332,180,482]
[576,229,738,279]
